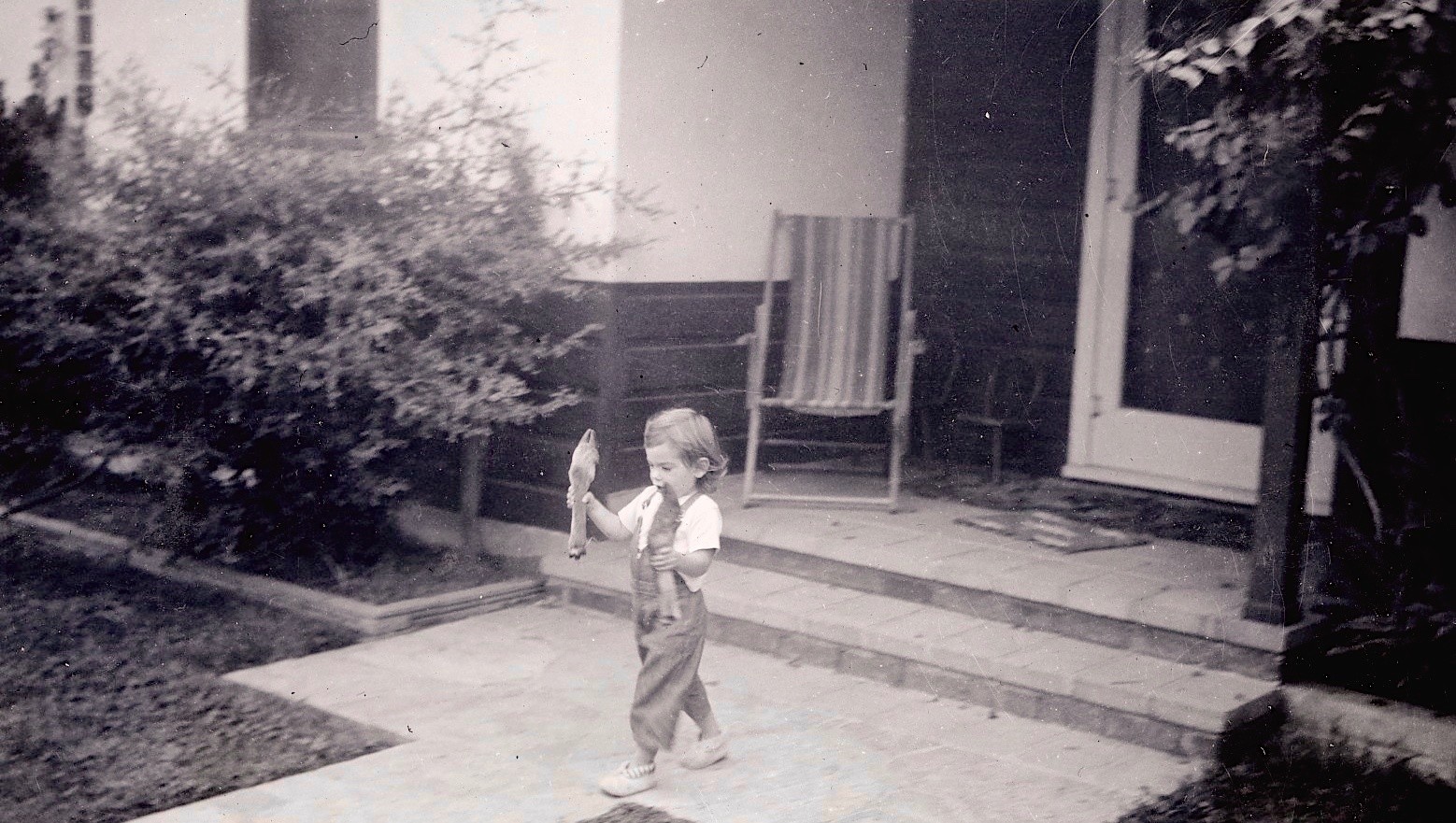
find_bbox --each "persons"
[565,407,731,798]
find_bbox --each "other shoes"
[680,729,727,770]
[600,761,657,796]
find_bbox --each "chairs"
[741,211,927,513]
[942,355,1049,484]
[858,344,962,474]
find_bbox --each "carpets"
[953,508,1150,555]
